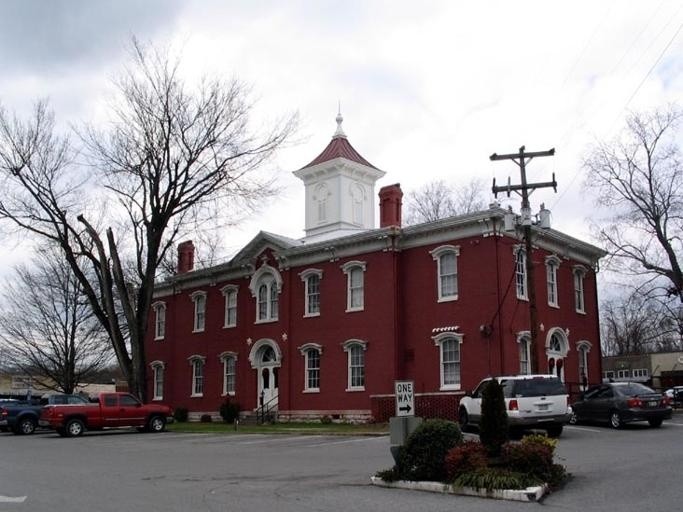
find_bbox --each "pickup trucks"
[38,391,174,438]
[0,394,90,435]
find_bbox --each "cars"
[663,386,683,409]
[0,399,21,432]
[566,382,672,430]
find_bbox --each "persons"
[580,372,587,391]
[479,377,508,421]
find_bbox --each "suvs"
[457,374,574,440]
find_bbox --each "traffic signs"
[394,379,416,418]
